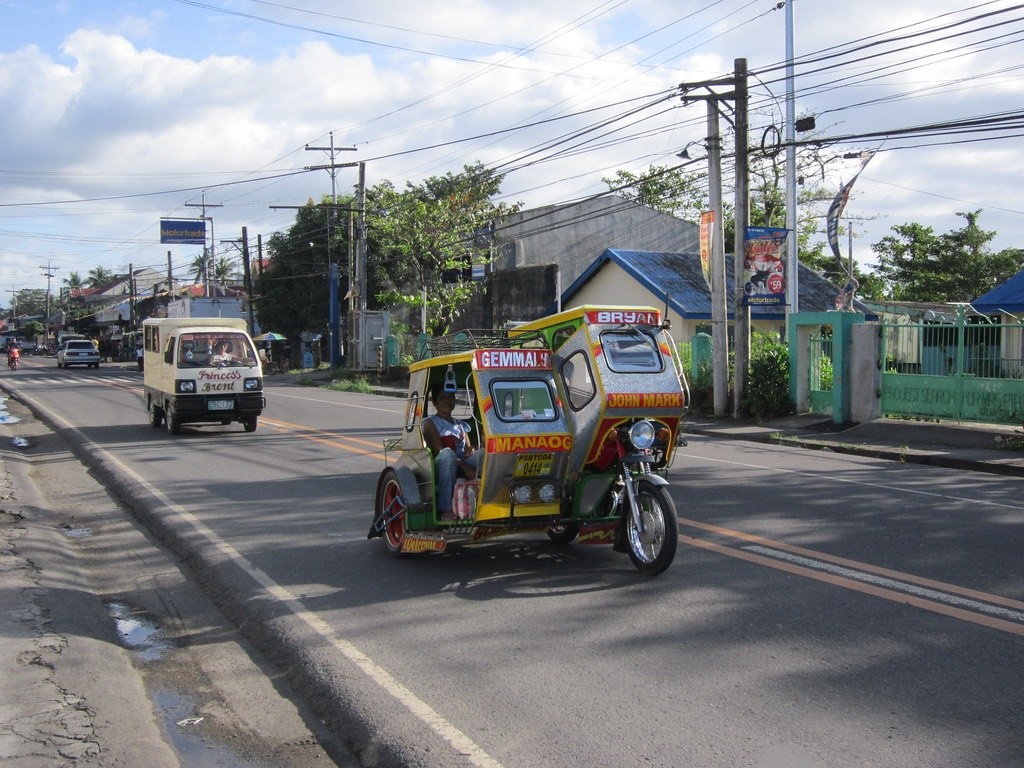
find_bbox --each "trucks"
[140,317,267,435]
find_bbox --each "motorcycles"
[6,346,21,371]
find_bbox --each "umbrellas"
[251,330,288,362]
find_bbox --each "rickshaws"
[370,300,691,575]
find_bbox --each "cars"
[57,339,101,370]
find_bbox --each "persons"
[92,337,99,350]
[207,339,241,367]
[135,345,143,374]
[421,385,487,520]
[8,336,19,349]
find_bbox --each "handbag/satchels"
[451,478,482,520]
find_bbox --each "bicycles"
[264,354,289,375]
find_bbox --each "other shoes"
[440,512,460,519]
